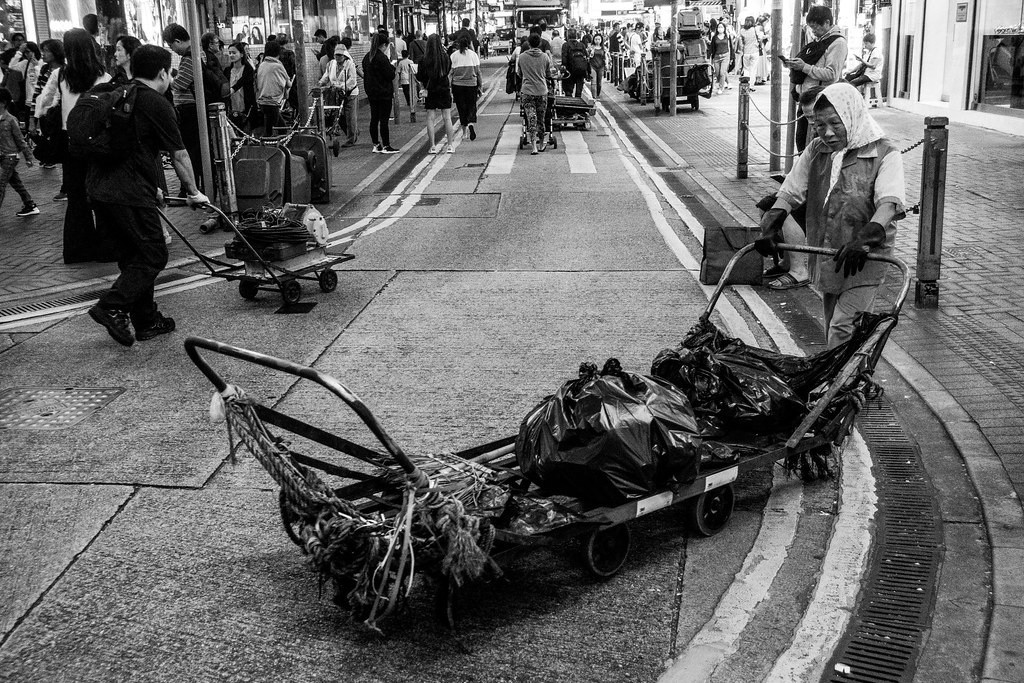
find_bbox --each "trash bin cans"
[651,45,685,98]
[231,143,315,215]
[677,8,705,36]
[684,39,712,94]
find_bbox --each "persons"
[114,36,172,244]
[788,6,848,157]
[755,83,906,352]
[609,21,663,78]
[843,33,884,87]
[703,13,771,94]
[34,28,116,263]
[30,39,67,201]
[162,23,207,206]
[87,45,210,348]
[994,37,1014,75]
[201,33,299,137]
[7,41,47,106]
[511,23,612,99]
[0,33,27,101]
[0,88,40,216]
[311,18,490,153]
[517,33,552,155]
[251,27,263,44]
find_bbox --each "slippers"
[530,144,547,155]
[769,272,810,290]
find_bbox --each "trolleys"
[298,85,347,158]
[659,63,715,113]
[155,195,355,306]
[515,62,592,149]
[182,239,912,612]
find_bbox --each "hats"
[334,44,350,57]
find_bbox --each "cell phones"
[778,55,788,65]
[390,60,397,65]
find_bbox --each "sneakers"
[16,205,41,216]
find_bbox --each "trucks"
[511,0,567,55]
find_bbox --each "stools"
[864,82,884,108]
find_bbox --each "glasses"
[25,50,32,54]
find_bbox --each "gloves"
[157,187,164,200]
[833,222,887,280]
[759,209,786,265]
[186,190,210,211]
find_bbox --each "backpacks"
[67,81,137,181]
[789,35,845,85]
[569,40,587,74]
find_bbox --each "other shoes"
[135,317,175,341]
[53,193,69,201]
[44,163,56,169]
[341,123,484,155]
[718,88,723,94]
[89,305,134,346]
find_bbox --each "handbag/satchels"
[758,45,764,56]
[846,64,867,82]
[701,225,791,285]
[419,89,428,98]
[728,54,735,73]
[591,56,603,69]
